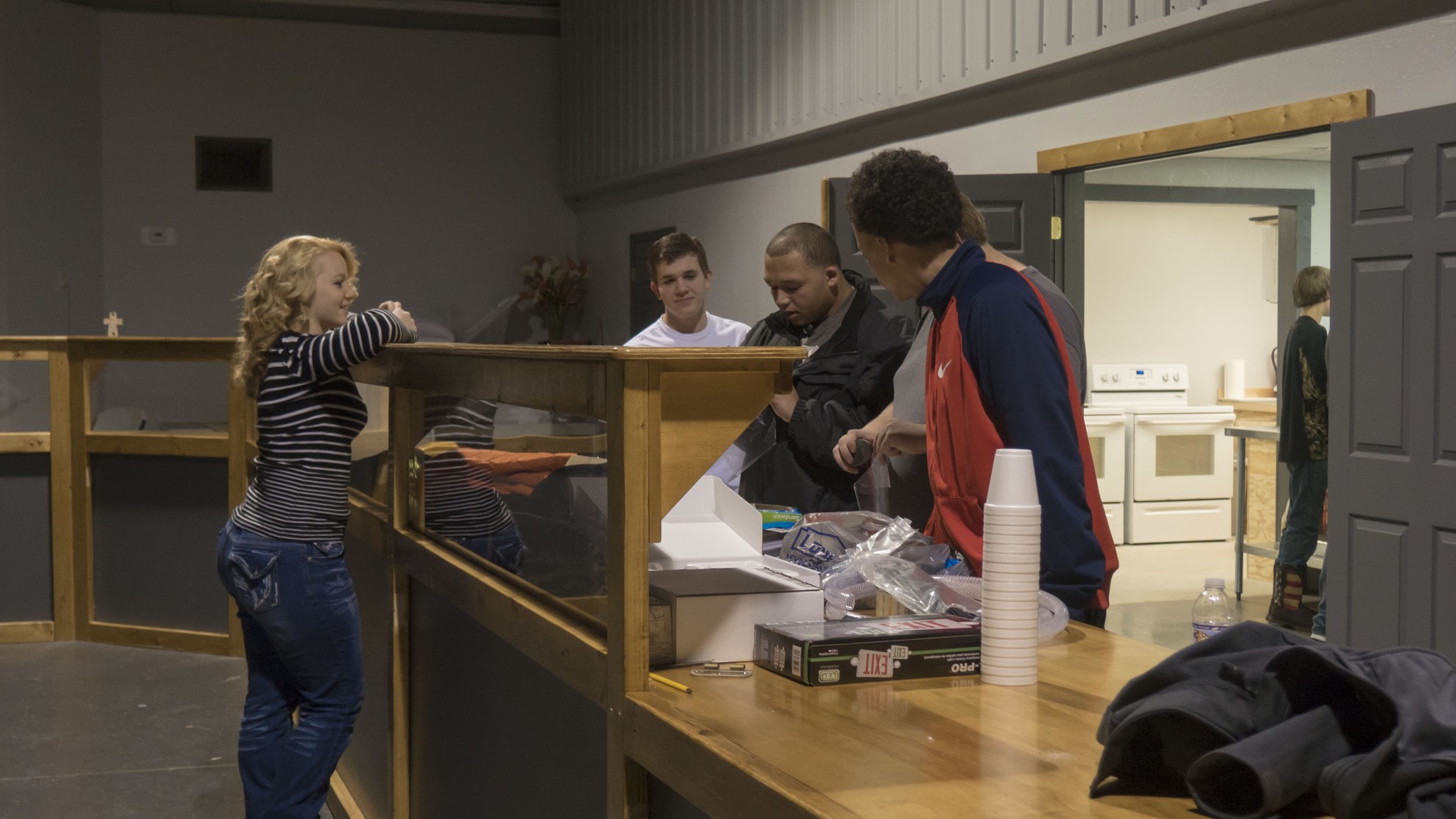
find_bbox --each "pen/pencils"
[648,672,693,693]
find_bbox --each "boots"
[1269,566,1318,633]
[1266,566,1278,621]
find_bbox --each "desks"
[1222,427,1327,599]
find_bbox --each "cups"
[980,448,1042,687]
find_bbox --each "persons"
[1310,542,1327,641]
[731,223,917,516]
[831,196,1086,535]
[425,391,525,576]
[1265,266,1331,632]
[622,233,755,495]
[216,235,418,819]
[844,148,1120,627]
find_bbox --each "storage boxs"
[649,473,825,664]
[754,611,992,686]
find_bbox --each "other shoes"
[1311,633,1326,641]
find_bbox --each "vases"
[546,306,565,340]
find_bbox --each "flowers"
[518,256,590,311]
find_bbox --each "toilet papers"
[1224,359,1245,399]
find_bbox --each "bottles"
[1191,578,1236,645]
